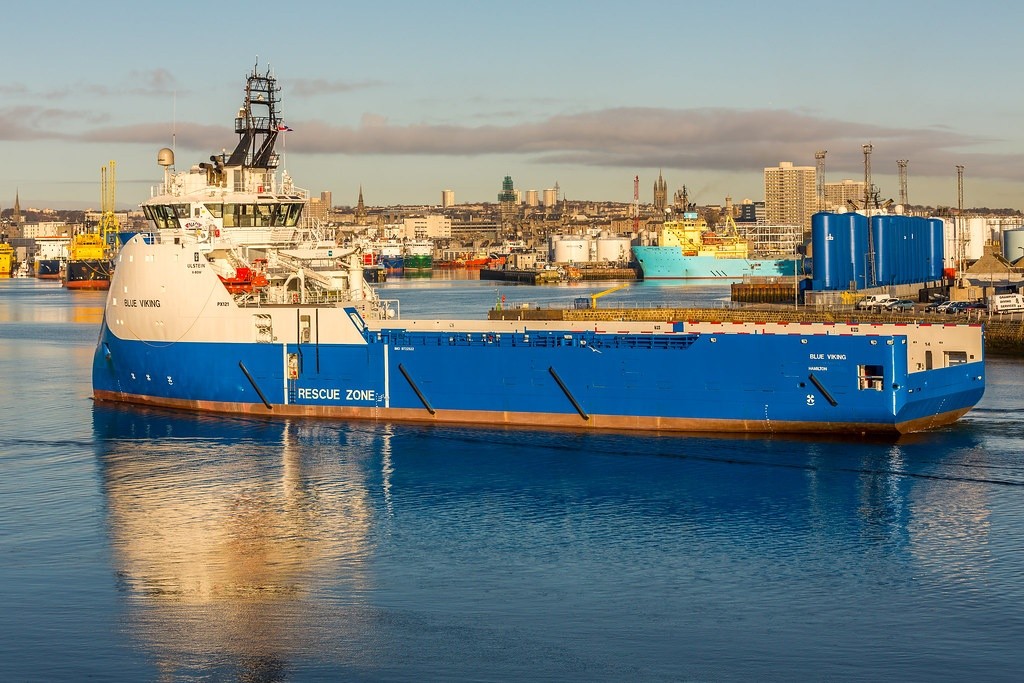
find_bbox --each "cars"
[925,301,987,314]
[886,300,915,312]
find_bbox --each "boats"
[631,213,812,278]
[535,266,583,282]
[38,260,60,279]
[66,259,110,290]
[92,56,985,435]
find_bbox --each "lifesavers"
[259,186,263,192]
[293,293,298,302]
[215,230,219,237]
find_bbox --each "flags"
[278,124,292,131]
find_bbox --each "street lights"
[859,252,876,311]
[793,233,797,310]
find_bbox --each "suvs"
[873,298,900,310]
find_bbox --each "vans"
[859,294,890,310]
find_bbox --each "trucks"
[988,293,1024,315]
